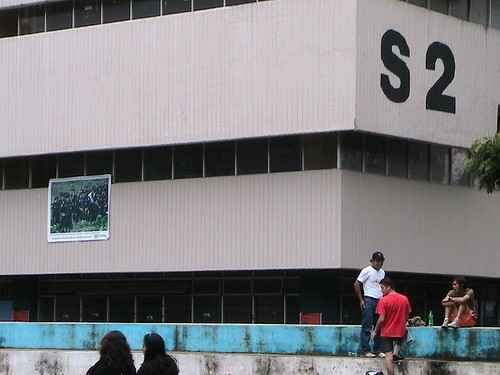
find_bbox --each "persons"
[86,331,136,375]
[353,252,386,358]
[442,277,478,328]
[371,277,411,375]
[136,333,179,375]
[51,179,108,233]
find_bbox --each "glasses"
[451,284,456,286]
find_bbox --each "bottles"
[348,352,362,357]
[428,310,433,327]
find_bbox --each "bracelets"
[448,296,453,301]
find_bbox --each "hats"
[370,252,385,263]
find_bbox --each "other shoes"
[365,353,376,358]
[441,318,449,328]
[448,317,459,328]
[378,353,385,358]
[393,345,404,360]
